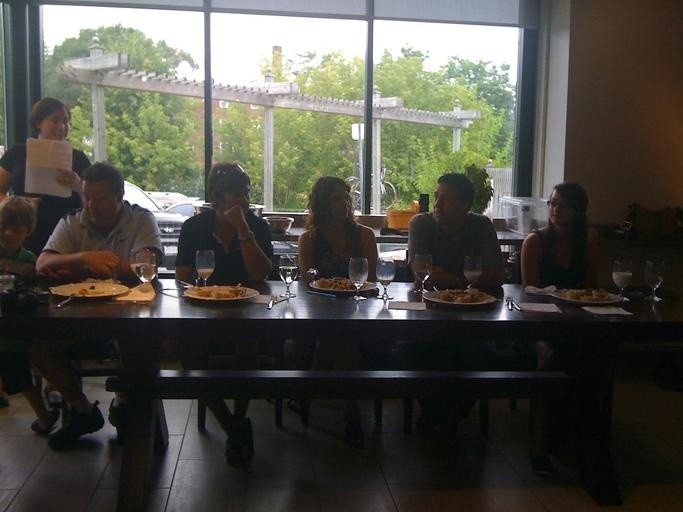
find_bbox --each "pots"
[248,203,263,221]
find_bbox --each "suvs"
[120,179,191,270]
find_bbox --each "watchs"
[238,230,255,241]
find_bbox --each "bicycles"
[342,165,397,210]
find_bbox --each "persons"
[173,162,274,466]
[405,172,508,439]
[29,161,165,452]
[1,98,91,260]
[0,194,62,436]
[515,181,615,477]
[297,175,379,450]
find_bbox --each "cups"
[0,274,15,292]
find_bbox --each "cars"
[146,191,206,218]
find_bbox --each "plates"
[50,282,129,300]
[422,289,496,306]
[551,289,621,306]
[184,285,260,302]
[309,280,378,293]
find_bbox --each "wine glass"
[278,298,297,319]
[348,257,369,301]
[195,250,216,287]
[278,255,299,298]
[350,301,366,319]
[377,300,392,320]
[612,258,633,301]
[129,248,149,285]
[413,253,432,294]
[644,259,665,303]
[135,251,158,285]
[376,256,396,300]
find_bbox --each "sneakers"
[226,418,254,467]
[108,398,131,443]
[530,449,553,474]
[346,422,364,447]
[48,400,104,450]
[31,409,59,434]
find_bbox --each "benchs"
[106,368,567,512]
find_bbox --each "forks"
[506,295,513,310]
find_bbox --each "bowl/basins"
[262,216,295,233]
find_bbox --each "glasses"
[547,201,567,208]
[213,163,246,177]
